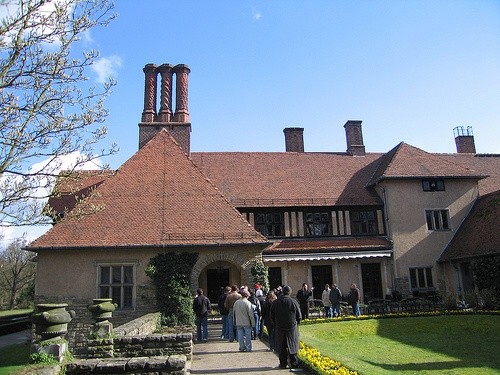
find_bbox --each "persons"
[349,284,360,317]
[193,288,212,341]
[218,283,342,371]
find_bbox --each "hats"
[255,284,260,289]
[240,289,251,297]
[282,285,292,295]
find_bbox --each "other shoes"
[203,339,209,342]
[270,347,275,352]
[290,363,298,368]
[279,364,289,369]
